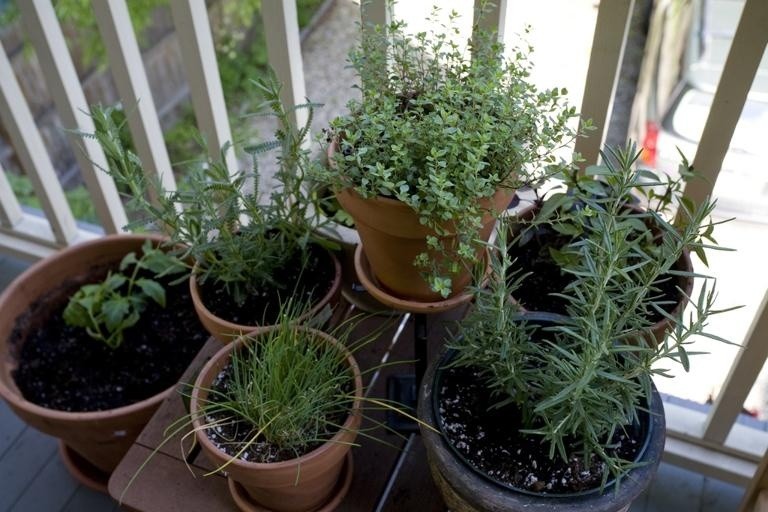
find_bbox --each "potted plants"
[75,70,345,346]
[325,1,597,314]
[413,136,745,510]
[180,293,433,510]
[0,232,210,499]
